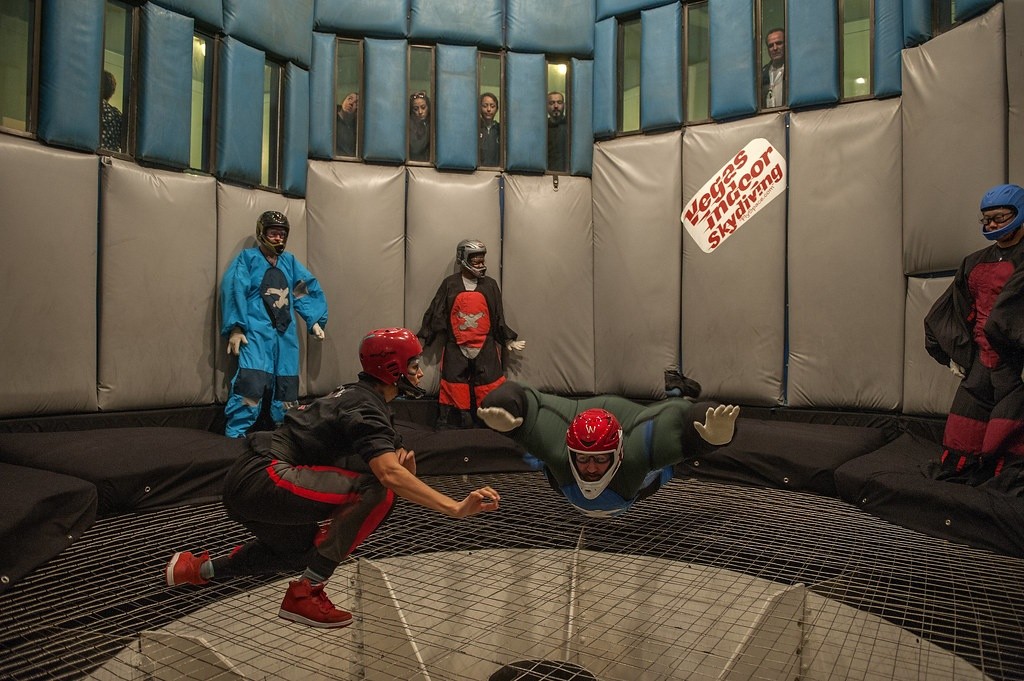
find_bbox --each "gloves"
[950,358,965,379]
[506,339,526,353]
[477,407,523,432]
[227,327,248,356]
[312,323,325,342]
[693,405,740,445]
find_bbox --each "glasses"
[407,357,420,375]
[266,231,286,239]
[575,453,612,464]
[469,257,484,265]
[980,211,1017,226]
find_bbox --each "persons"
[101,71,122,152]
[924,185,1024,481]
[221,211,329,438]
[165,327,500,628]
[480,93,499,167]
[415,239,526,430]
[410,93,429,161]
[477,370,740,518]
[548,92,567,172]
[337,91,357,156]
[763,28,782,108]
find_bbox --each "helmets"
[566,408,624,500]
[456,238,487,279]
[256,211,290,256]
[359,327,427,400]
[980,184,1024,241]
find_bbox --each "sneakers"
[664,370,701,399]
[166,550,209,586]
[278,578,353,628]
